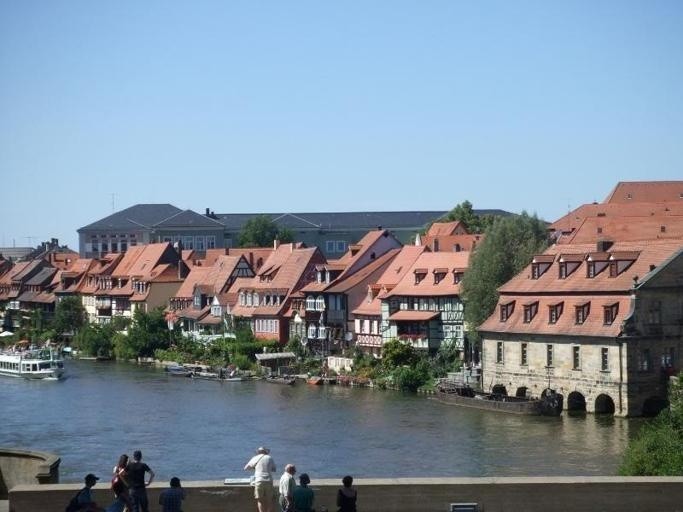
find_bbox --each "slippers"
[66,491,81,511]
[111,466,126,494]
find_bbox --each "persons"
[66,474,103,511]
[118,450,155,511]
[110,453,132,511]
[278,463,296,512]
[243,445,277,511]
[292,472,315,511]
[158,476,186,511]
[336,475,356,511]
[7,343,39,355]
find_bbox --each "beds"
[18,339,28,344]
[0,330,15,340]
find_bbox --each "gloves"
[305,377,324,385]
[167,361,249,381]
[264,374,295,385]
[436,381,562,417]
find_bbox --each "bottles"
[84,473,100,482]
[254,446,271,454]
